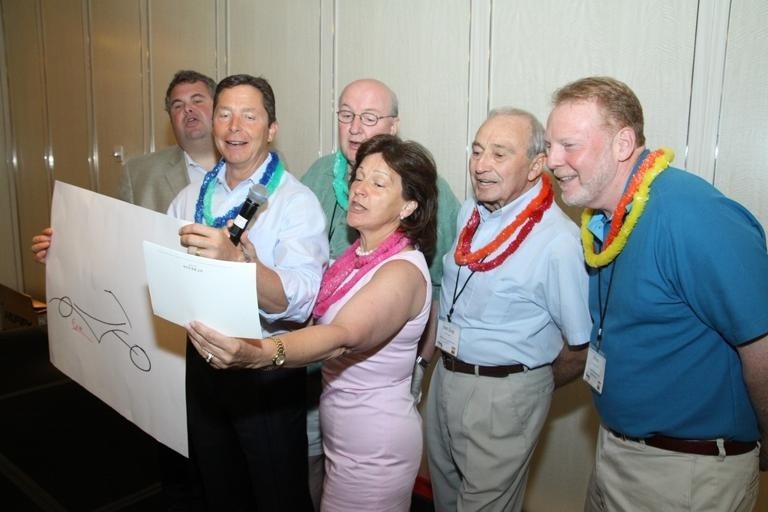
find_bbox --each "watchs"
[236,245,253,264]
[417,355,427,368]
[266,336,286,368]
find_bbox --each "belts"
[442,353,553,378]
[611,431,757,455]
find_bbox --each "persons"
[300,77,458,408]
[425,108,593,512]
[33,74,330,512]
[543,77,768,512]
[186,133,440,512]
[113,69,219,217]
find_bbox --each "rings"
[206,352,213,363]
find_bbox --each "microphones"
[229,184,268,247]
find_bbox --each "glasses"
[337,111,396,126]
